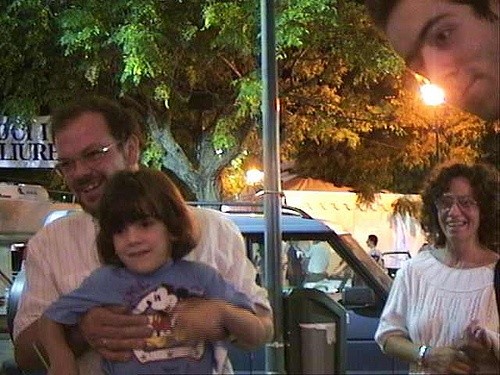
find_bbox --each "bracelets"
[417,345,431,366]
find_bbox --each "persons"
[374,161,500,375]
[285,234,383,289]
[12,94,275,375]
[38,170,267,375]
[367,0,500,122]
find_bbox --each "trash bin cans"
[281,285,348,375]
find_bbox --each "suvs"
[6,201,411,375]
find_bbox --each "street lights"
[419,83,445,164]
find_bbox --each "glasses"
[435,194,478,208]
[55,141,120,176]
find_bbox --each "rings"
[102,338,108,348]
